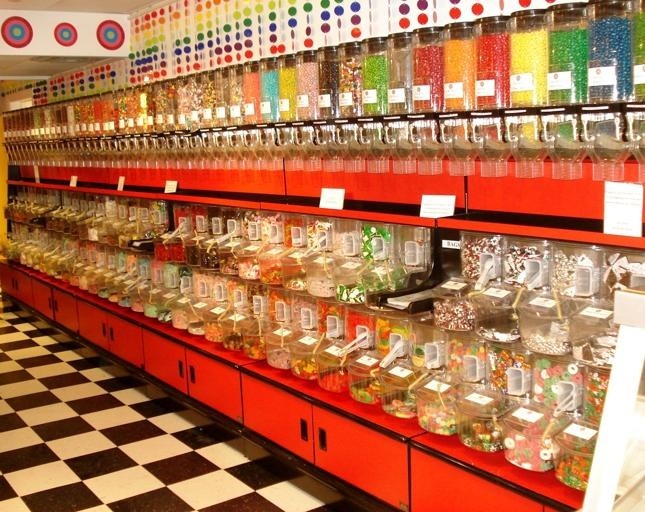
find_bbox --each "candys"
[581,367,610,426]
[446,335,485,376]
[530,358,582,412]
[416,389,461,436]
[486,348,530,394]
[501,413,553,472]
[553,426,598,492]
[461,420,501,453]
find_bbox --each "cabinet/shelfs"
[240,369,411,512]
[0,258,33,311]
[77,296,143,379]
[32,273,77,337]
[267,169,468,239]
[435,167,645,261]
[143,326,242,428]
[412,438,584,512]
[5,164,287,223]
